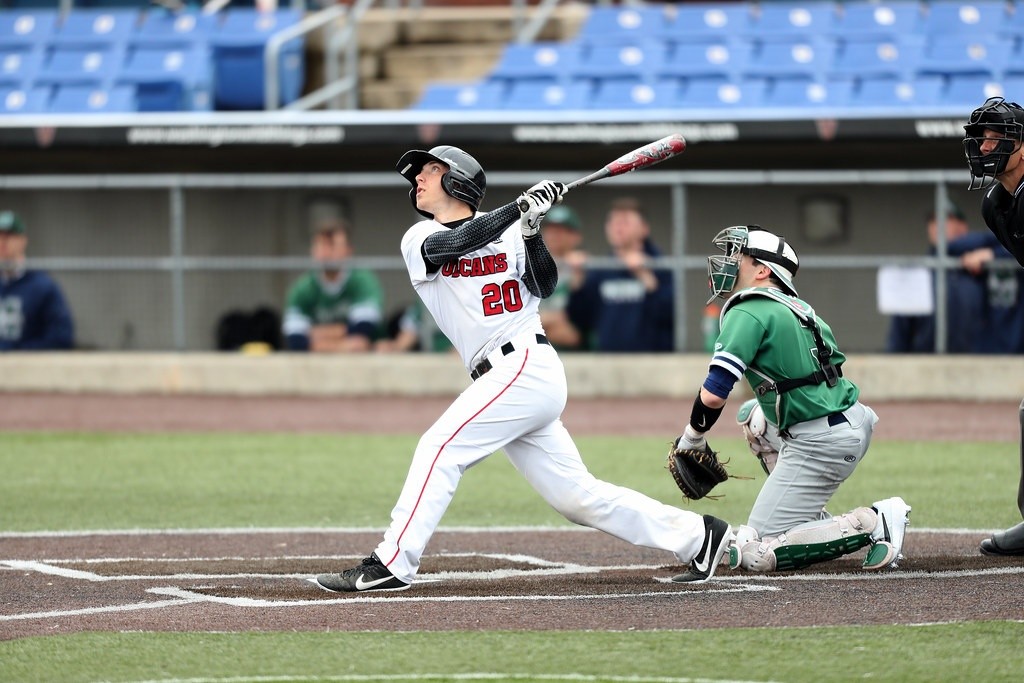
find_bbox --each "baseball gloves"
[668,435,729,500]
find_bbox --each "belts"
[827,410,849,426]
[470,333,548,383]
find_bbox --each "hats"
[0,212,28,232]
[543,203,582,228]
[306,202,347,232]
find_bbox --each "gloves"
[517,180,569,239]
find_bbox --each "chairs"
[0,0,306,115]
[406,0,1024,107]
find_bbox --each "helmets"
[397,145,488,220]
[730,225,803,296]
[965,97,1024,141]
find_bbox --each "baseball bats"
[520,133,686,212]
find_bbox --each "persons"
[378,297,458,353]
[0,210,77,351]
[281,220,386,350]
[963,96,1024,557]
[314,146,737,590]
[539,204,589,350]
[888,210,1024,353]
[668,226,913,572]
[569,196,674,352]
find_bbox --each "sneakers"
[670,515,730,584]
[867,496,912,570]
[313,552,412,594]
[978,522,1024,554]
